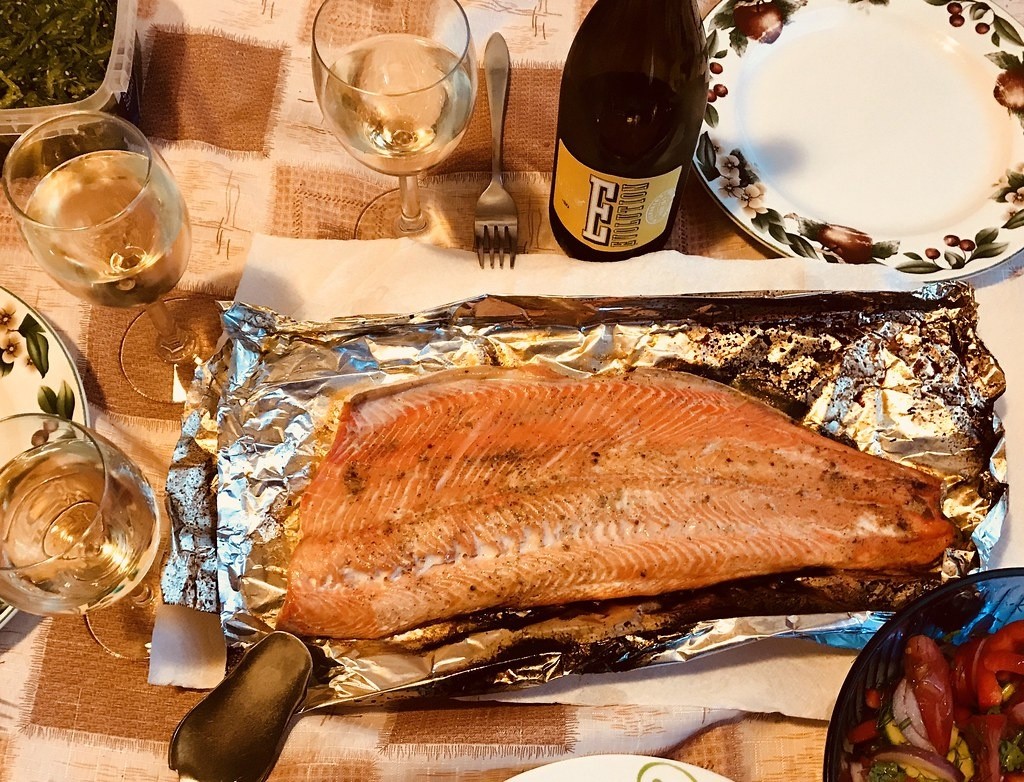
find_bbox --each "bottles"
[547,2,710,260]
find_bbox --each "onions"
[849,676,966,782]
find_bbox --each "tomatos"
[902,619,1024,782]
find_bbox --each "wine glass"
[1,111,232,404]
[311,0,469,257]
[1,412,197,662]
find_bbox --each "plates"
[695,0,1024,282]
[499,753,741,782]
[1,285,93,635]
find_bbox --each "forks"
[475,29,521,270]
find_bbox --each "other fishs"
[277,362,954,641]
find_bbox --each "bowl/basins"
[821,567,1024,782]
[1,0,144,169]
